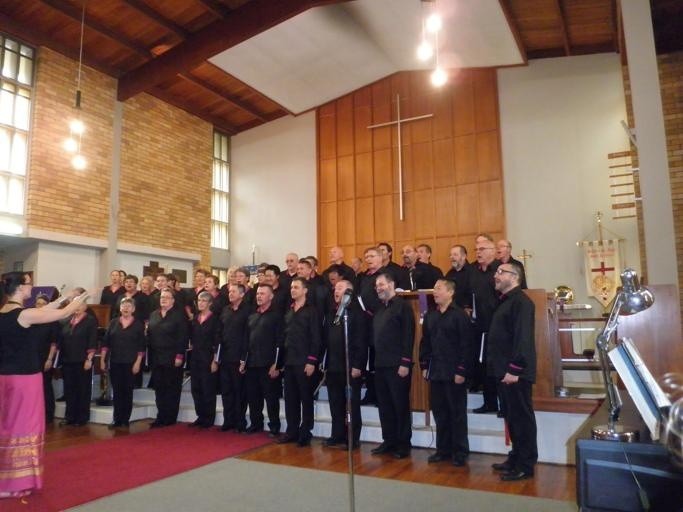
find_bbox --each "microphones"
[334,288,354,326]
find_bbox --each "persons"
[445,234,527,418]
[484,264,538,481]
[352,242,416,460]
[33,288,99,428]
[274,245,362,451]
[400,244,443,291]
[0,271,94,506]
[419,277,471,467]
[99,268,186,430]
[182,261,285,437]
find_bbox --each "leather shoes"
[0,490,31,498]
[370,442,534,481]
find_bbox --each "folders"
[606,336,672,443]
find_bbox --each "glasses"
[21,279,33,286]
